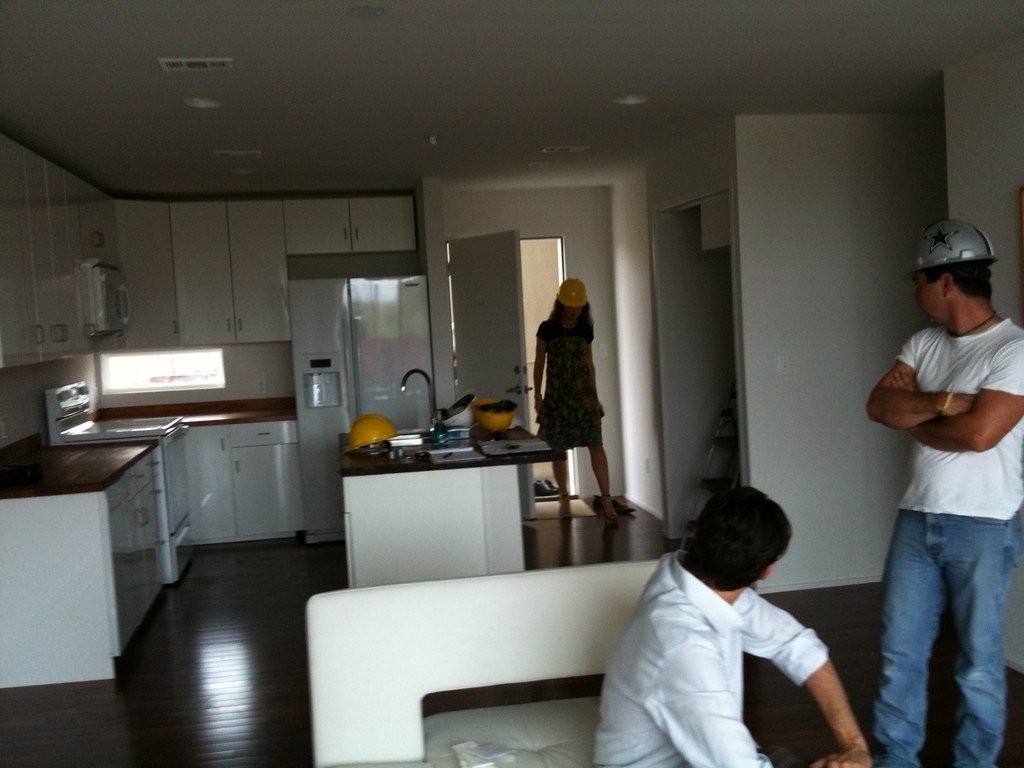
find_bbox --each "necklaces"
[947,310,997,337]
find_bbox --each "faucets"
[400,368,437,432]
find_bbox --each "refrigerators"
[287,274,435,545]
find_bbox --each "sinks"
[386,433,421,440]
[389,438,424,447]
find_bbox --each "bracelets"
[534,394,542,398]
[941,392,954,416]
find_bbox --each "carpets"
[522,498,597,521]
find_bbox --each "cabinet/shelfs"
[169,200,293,347]
[0,134,125,369]
[0,452,167,689]
[115,199,179,351]
[282,197,417,257]
[183,419,306,553]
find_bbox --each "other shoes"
[602,508,618,528]
[560,501,572,518]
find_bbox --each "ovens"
[152,426,196,584]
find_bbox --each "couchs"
[302,560,664,768]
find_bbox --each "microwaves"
[92,267,125,332]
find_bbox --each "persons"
[866,220,1024,768]
[533,279,619,521]
[594,485,873,768]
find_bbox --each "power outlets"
[645,458,650,475]
[255,379,267,394]
[0,415,8,440]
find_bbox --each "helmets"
[907,219,998,274]
[557,279,588,307]
[343,414,398,454]
[442,394,477,427]
[472,399,517,433]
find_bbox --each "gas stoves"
[46,379,183,442]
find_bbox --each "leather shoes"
[593,495,637,514]
[535,479,560,495]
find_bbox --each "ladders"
[675,382,740,551]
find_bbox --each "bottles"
[430,411,448,443]
